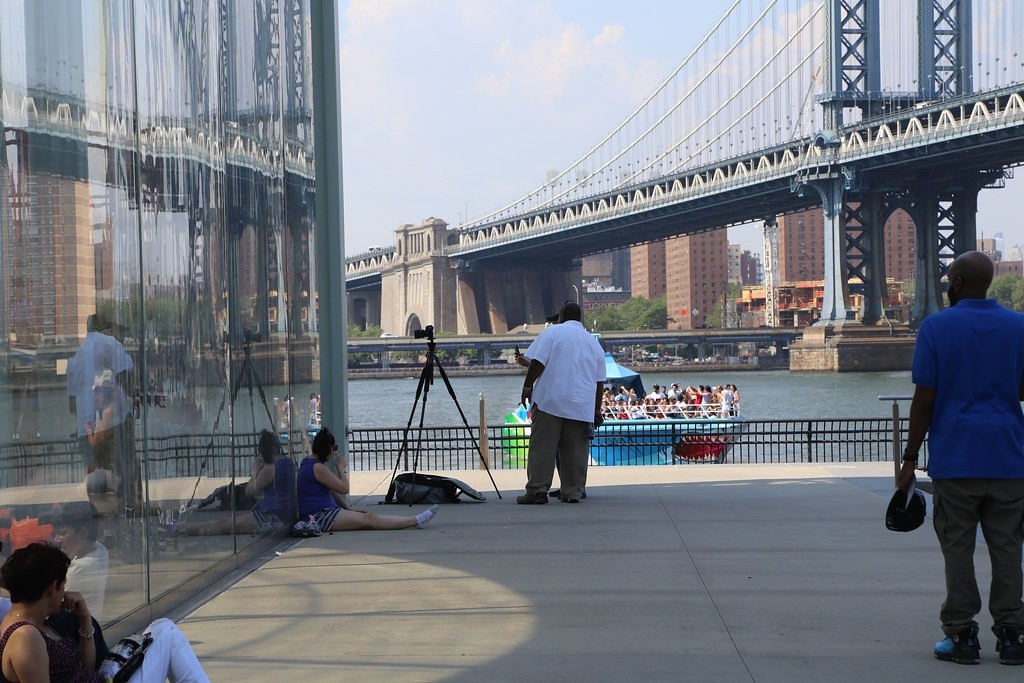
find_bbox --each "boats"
[499,393,748,466]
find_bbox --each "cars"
[758,325,772,328]
[379,333,398,338]
[693,323,715,329]
[516,330,529,334]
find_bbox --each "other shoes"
[122,502,162,517]
[558,492,586,500]
[549,489,560,497]
[517,493,549,504]
[561,498,580,503]
[161,508,177,537]
[416,504,439,529]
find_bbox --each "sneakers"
[991,625,1024,664]
[934,620,981,664]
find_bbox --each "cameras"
[414,325,434,339]
[243,330,262,344]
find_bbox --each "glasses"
[333,445,339,451]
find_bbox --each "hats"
[114,324,130,332]
[654,383,659,387]
[886,472,926,532]
[546,301,571,321]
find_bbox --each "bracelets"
[78,625,95,638]
[903,447,919,464]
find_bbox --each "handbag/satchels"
[97,633,154,683]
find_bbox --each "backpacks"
[199,482,249,511]
[385,469,486,502]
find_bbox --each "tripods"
[386,338,503,508]
[185,344,286,510]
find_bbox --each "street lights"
[572,284,579,305]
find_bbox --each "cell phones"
[514,345,521,357]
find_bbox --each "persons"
[0,501,110,624]
[595,384,740,419]
[166,396,297,536]
[513,302,602,504]
[67,314,159,517]
[306,391,324,432]
[896,252,1024,664]
[296,428,441,531]
[0,543,213,683]
